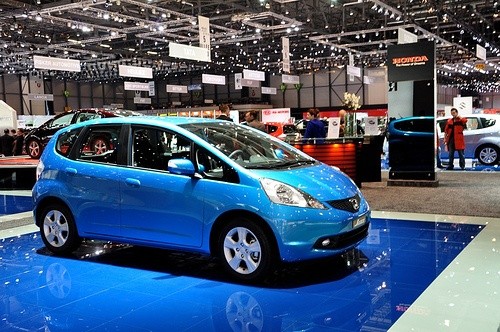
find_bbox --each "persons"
[302,109,326,145]
[437,111,446,168]
[240,110,266,161]
[0,127,24,157]
[210,104,235,161]
[444,108,467,170]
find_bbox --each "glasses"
[451,112,456,114]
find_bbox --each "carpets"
[358,170,500,217]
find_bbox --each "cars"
[24,107,148,160]
[31,115,371,286]
[277,119,343,138]
[437,113,500,166]
[261,121,284,139]
[388,116,434,157]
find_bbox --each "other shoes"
[446,165,454,170]
[460,166,465,170]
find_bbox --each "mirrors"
[388,80,435,170]
